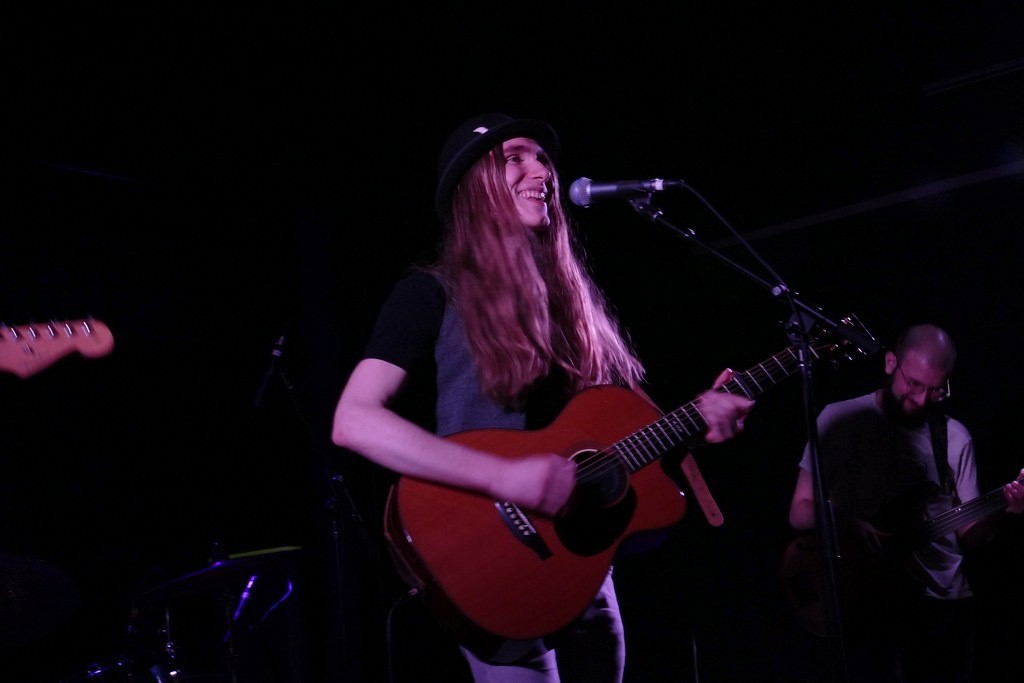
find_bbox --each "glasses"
[897,362,950,406]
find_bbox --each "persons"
[330,111,754,683]
[787,325,1023,681]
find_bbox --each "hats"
[435,114,560,214]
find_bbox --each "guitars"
[1,319,115,376]
[382,312,864,666]
[779,472,1024,643]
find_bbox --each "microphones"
[253,334,286,406]
[569,176,686,209]
